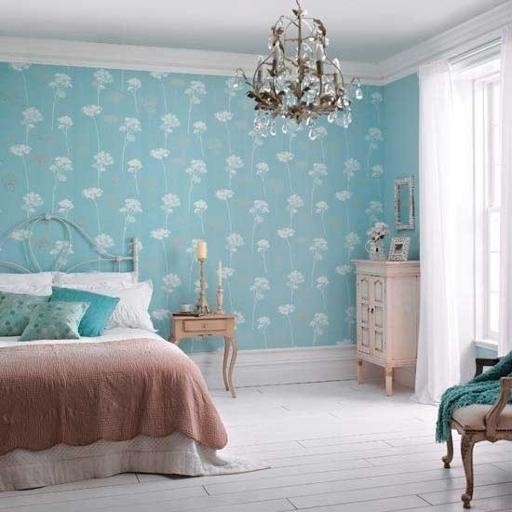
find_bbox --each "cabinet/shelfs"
[349,257,421,398]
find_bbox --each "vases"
[365,239,387,260]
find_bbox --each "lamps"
[234,2,367,137]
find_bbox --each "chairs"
[436,351,512,511]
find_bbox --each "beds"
[1,212,228,494]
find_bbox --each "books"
[173,311,205,317]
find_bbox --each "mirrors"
[393,173,417,232]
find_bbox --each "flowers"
[365,220,390,242]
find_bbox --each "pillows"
[2,270,163,343]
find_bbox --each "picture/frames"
[386,236,412,263]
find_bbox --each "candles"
[216,259,225,285]
[195,239,209,260]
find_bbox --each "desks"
[169,310,238,401]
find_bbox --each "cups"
[180,304,193,313]
